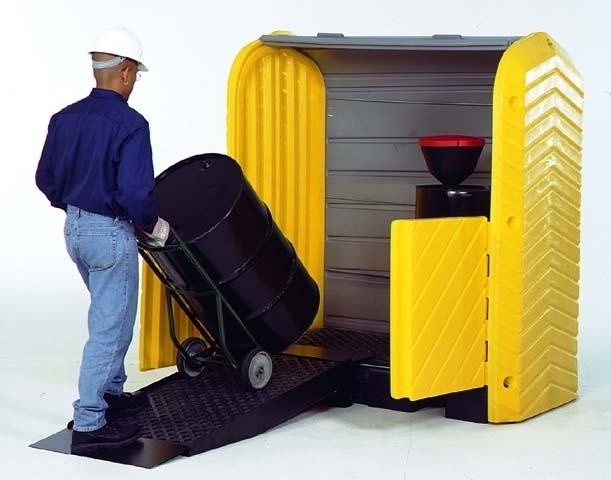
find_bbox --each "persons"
[35,25,171,449]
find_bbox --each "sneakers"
[71,422,142,453]
[103,392,145,414]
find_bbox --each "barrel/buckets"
[134,154,321,356]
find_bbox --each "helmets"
[89,27,148,71]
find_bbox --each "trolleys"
[136,211,279,393]
